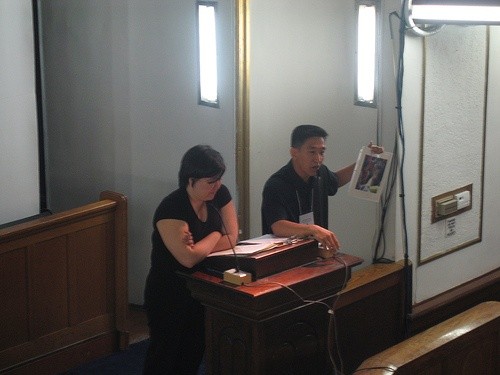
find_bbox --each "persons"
[261,125,383,253]
[143,144,239,375]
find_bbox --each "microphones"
[206,202,240,273]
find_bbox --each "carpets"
[59,336,207,375]
